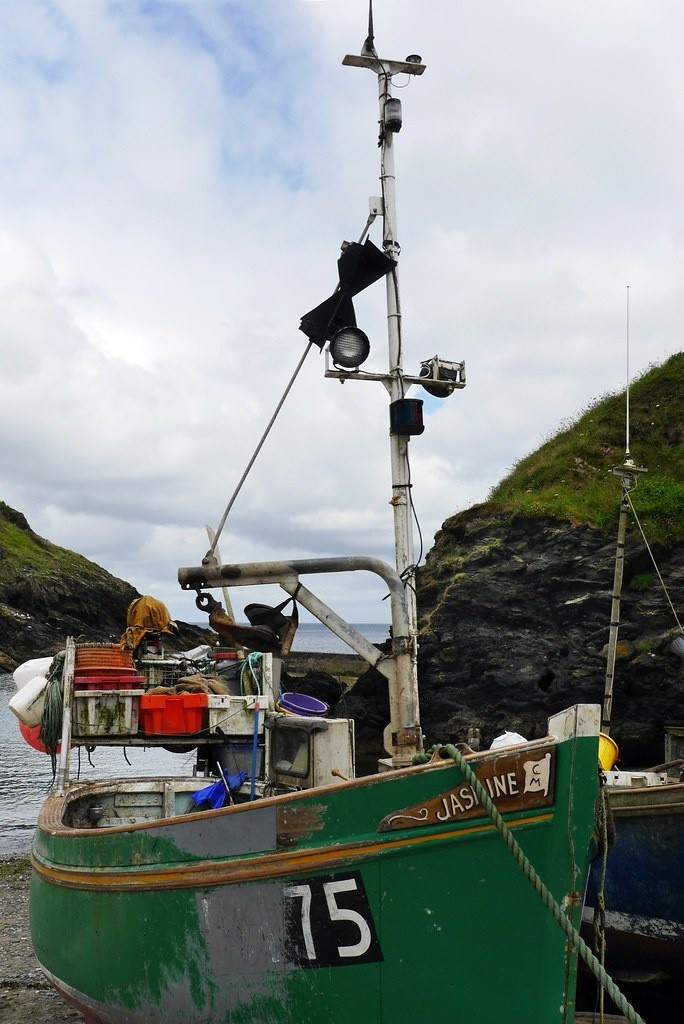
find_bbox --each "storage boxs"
[74,649,268,737]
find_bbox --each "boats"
[488,283,684,1024]
[27,2,606,1024]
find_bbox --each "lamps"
[330,327,370,367]
[419,361,457,397]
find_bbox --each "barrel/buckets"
[275,692,328,717]
[7,664,59,728]
[12,657,55,690]
[18,718,76,753]
[598,732,619,771]
